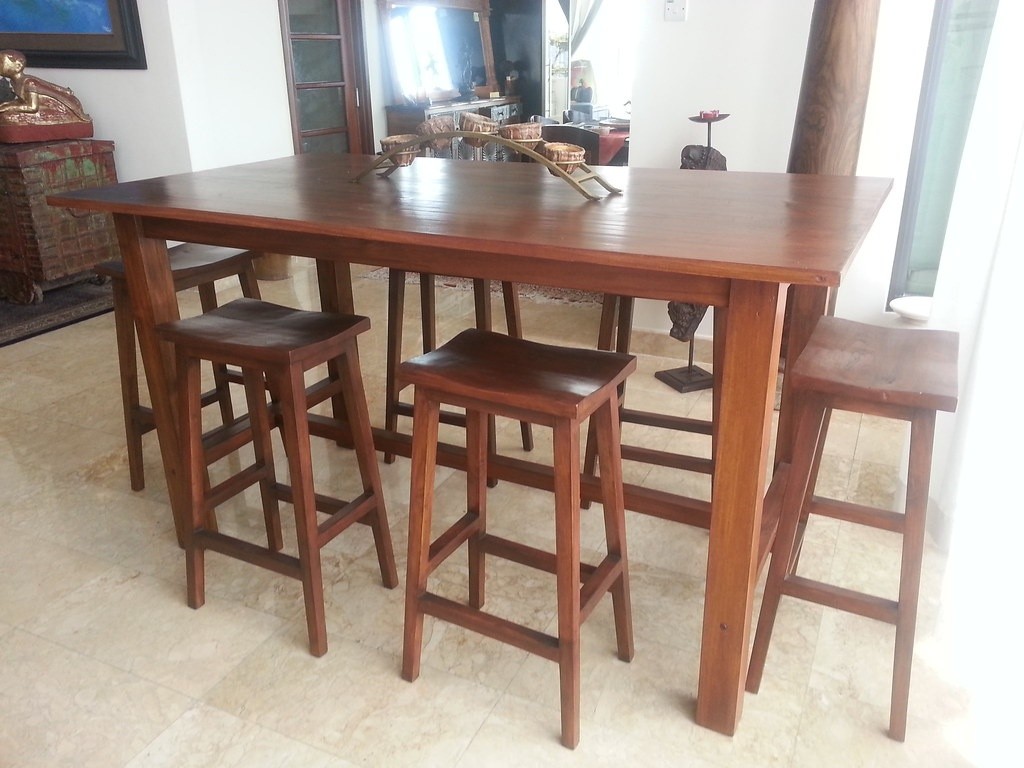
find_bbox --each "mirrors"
[378,0,498,104]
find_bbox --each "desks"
[42,152,897,738]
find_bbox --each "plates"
[601,119,629,128]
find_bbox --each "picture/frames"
[0,0,148,71]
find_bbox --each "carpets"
[0,276,116,350]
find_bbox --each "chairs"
[563,110,591,124]
[541,125,600,165]
[528,115,559,123]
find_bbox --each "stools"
[93,241,958,748]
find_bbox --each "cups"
[544,143,585,176]
[380,135,419,167]
[460,112,499,147]
[499,123,542,151]
[416,115,454,152]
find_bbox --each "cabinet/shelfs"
[384,95,526,161]
